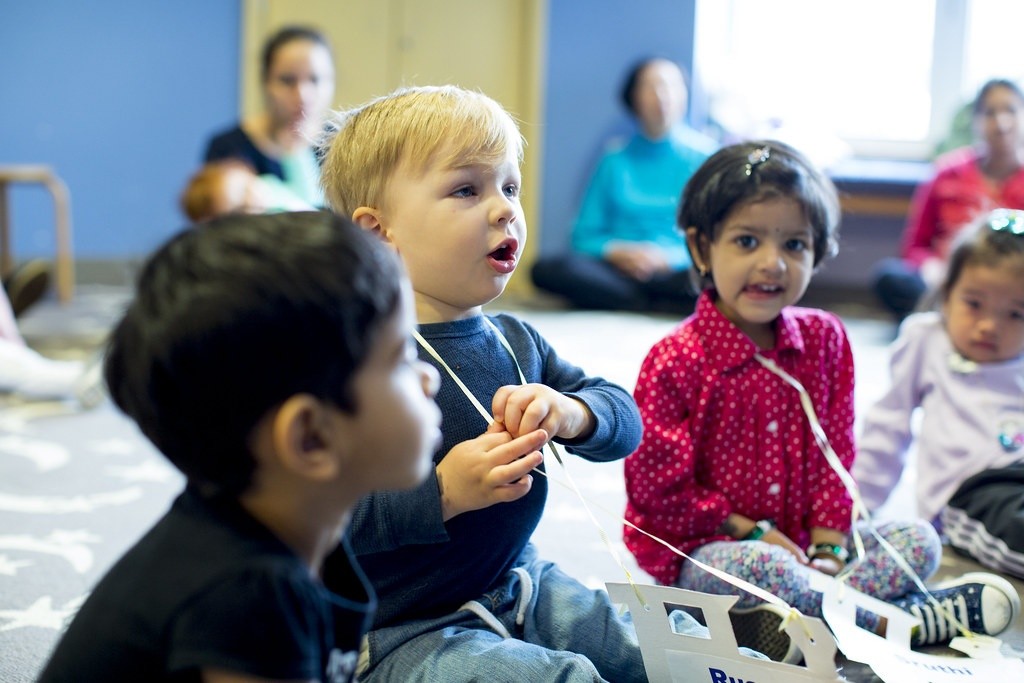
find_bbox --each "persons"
[182,26,340,225]
[848,207,1024,581]
[316,84,772,683]
[874,79,1024,321]
[528,55,720,316]
[622,139,1019,648]
[36,207,443,683]
[0,284,103,404]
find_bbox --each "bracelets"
[740,518,777,540]
[806,542,853,564]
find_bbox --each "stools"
[0,163,81,349]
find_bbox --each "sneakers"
[885,572,1020,649]
[699,602,805,667]
